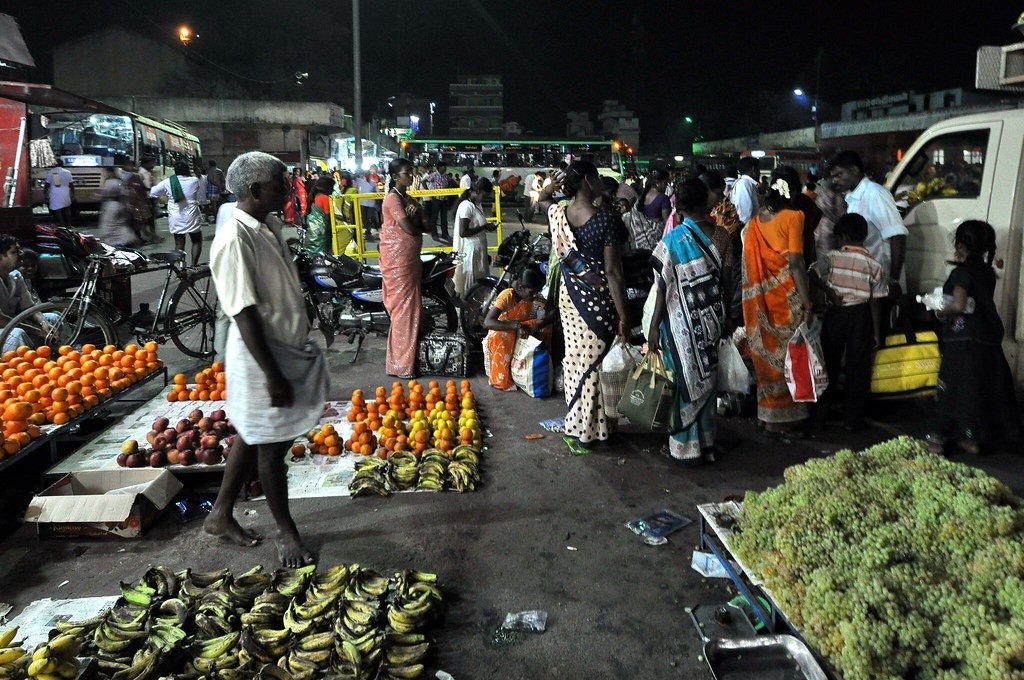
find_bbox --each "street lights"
[684,116,701,142]
[794,88,824,152]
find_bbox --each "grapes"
[721,434,1024,680]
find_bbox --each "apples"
[117,408,237,468]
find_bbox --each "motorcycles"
[284,223,464,362]
[462,207,553,346]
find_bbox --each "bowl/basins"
[730,595,771,631]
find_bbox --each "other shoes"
[765,422,809,439]
[673,458,702,467]
[704,447,717,461]
[598,440,609,448]
[953,438,981,457]
[923,432,947,455]
[577,438,593,449]
[396,374,415,378]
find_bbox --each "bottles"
[916,293,975,313]
[627,288,649,299]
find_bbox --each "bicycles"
[0,251,222,363]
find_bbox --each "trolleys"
[36,252,133,321]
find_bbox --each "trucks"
[883,41,1024,385]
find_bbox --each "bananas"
[346,445,483,500]
[908,177,960,204]
[0,562,443,680]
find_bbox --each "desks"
[0,365,169,476]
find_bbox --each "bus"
[29,108,205,215]
[622,160,649,176]
[692,153,733,178]
[738,148,829,188]
[399,137,633,202]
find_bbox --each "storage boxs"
[23,468,185,543]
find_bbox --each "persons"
[378,150,1016,460]
[194,159,383,230]
[96,156,165,249]
[44,159,75,228]
[0,235,73,354]
[150,162,203,265]
[203,150,328,566]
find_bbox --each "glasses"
[395,168,413,174]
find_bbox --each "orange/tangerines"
[165,360,226,402]
[0,341,163,460]
[292,379,481,456]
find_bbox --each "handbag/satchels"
[416,326,471,378]
[870,303,940,399]
[333,253,364,275]
[598,339,643,418]
[623,248,650,289]
[511,334,554,398]
[719,335,751,394]
[785,315,829,402]
[617,349,674,433]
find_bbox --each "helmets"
[103,179,124,197]
